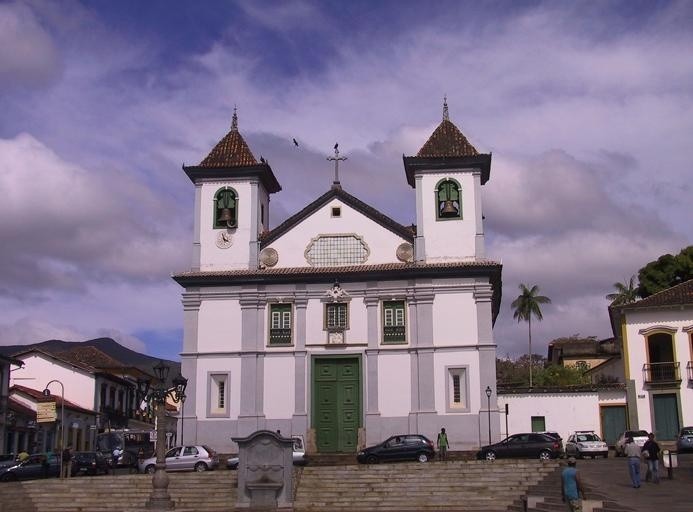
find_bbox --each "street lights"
[137,359,189,512]
[486,386,492,445]
[43,380,64,480]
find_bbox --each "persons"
[62,443,74,479]
[560,457,587,512]
[111,446,120,464]
[437,428,449,461]
[624,433,660,487]
[139,447,145,459]
[18,449,30,463]
[396,437,401,444]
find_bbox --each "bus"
[96,431,155,468]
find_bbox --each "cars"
[478,431,564,460]
[0,450,108,481]
[356,434,436,464]
[616,430,651,459]
[566,431,609,459]
[227,435,306,470]
[674,427,693,453]
[138,445,218,474]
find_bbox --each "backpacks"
[63,448,71,462]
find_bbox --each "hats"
[567,457,576,464]
